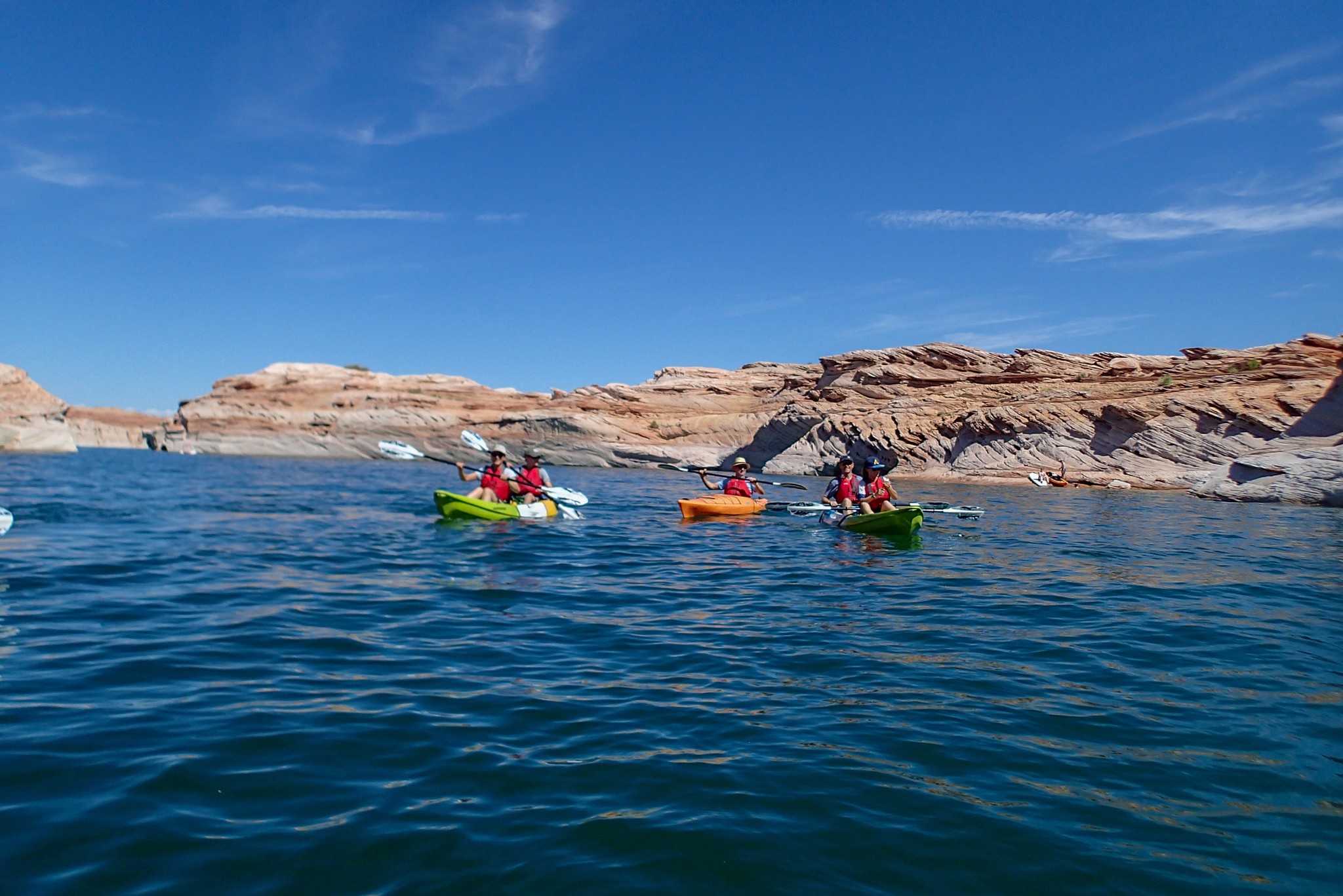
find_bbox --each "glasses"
[491,452,504,458]
[841,461,852,466]
[867,467,881,471]
[530,456,540,459]
[735,465,747,470]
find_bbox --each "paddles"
[787,502,986,520]
[0,506,14,538]
[379,439,589,506]
[459,429,585,518]
[656,462,810,489]
[764,502,949,513]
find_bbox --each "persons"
[1059,459,1066,480]
[1038,468,1051,484]
[822,455,898,517]
[699,457,764,498]
[456,444,553,505]
[1075,484,1079,488]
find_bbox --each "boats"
[1029,472,1047,487]
[1044,470,1069,488]
[677,493,774,520]
[818,503,925,539]
[431,488,559,524]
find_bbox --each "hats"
[491,444,506,457]
[863,456,885,469]
[839,455,854,463]
[523,447,544,458]
[731,457,751,471]
